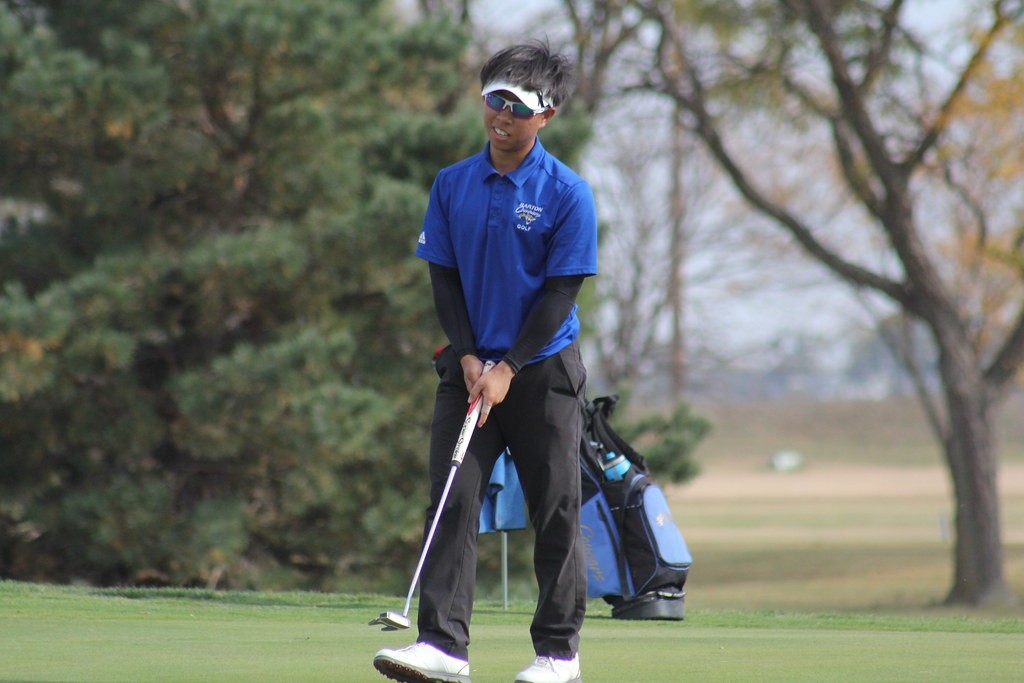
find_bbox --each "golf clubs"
[367,359,496,633]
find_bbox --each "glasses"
[480,90,547,121]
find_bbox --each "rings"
[482,405,491,414]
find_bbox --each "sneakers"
[513,650,582,683]
[373,641,471,683]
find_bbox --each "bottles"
[602,451,632,481]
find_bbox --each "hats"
[479,74,554,114]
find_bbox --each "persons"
[374,32,599,683]
